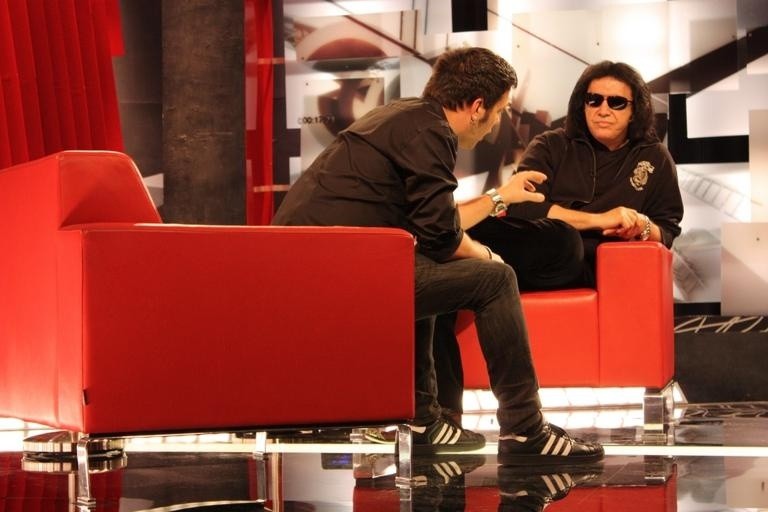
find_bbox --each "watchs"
[640,214,650,241]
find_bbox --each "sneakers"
[395,454,486,489]
[412,413,485,448]
[498,464,603,510]
[497,423,604,463]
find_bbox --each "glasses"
[583,93,633,110]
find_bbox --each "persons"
[270,47,604,461]
[365,60,685,441]
[405,461,604,512]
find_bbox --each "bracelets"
[485,245,494,261]
[486,188,508,217]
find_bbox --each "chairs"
[448,238,677,435]
[1,149,419,510]
